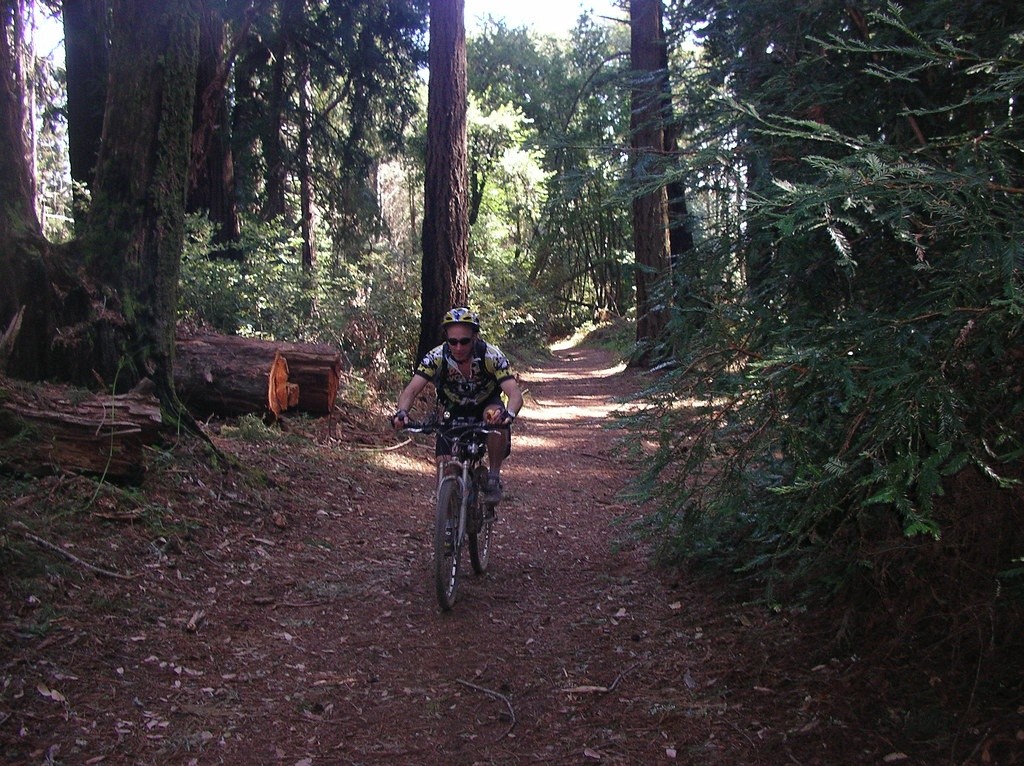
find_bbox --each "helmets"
[441,307,480,332]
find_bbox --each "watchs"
[506,410,515,421]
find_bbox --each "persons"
[394,308,524,554]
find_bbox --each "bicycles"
[397,412,501,610]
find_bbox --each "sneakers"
[443,526,458,555]
[482,477,502,504]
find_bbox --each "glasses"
[446,337,474,346]
